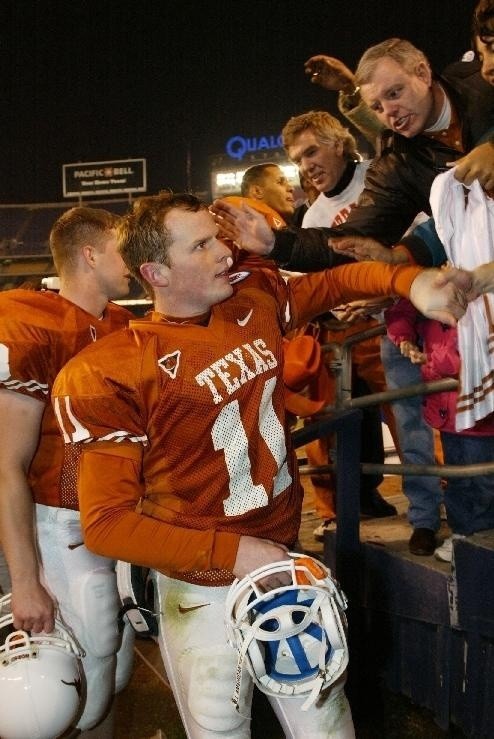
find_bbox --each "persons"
[1,205,140,738]
[205,0,493,560]
[48,186,466,736]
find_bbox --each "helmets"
[224,552,349,699]
[0,644,87,739]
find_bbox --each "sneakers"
[408,527,467,563]
[360,488,397,517]
[313,518,337,539]
